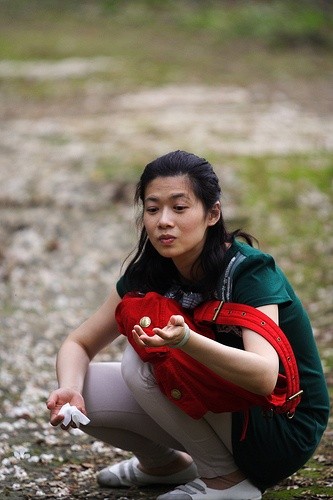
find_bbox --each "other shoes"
[95,453,200,488]
[156,475,263,500]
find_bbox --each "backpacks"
[114,290,304,421]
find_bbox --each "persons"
[46,151,330,500]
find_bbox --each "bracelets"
[169,323,190,348]
